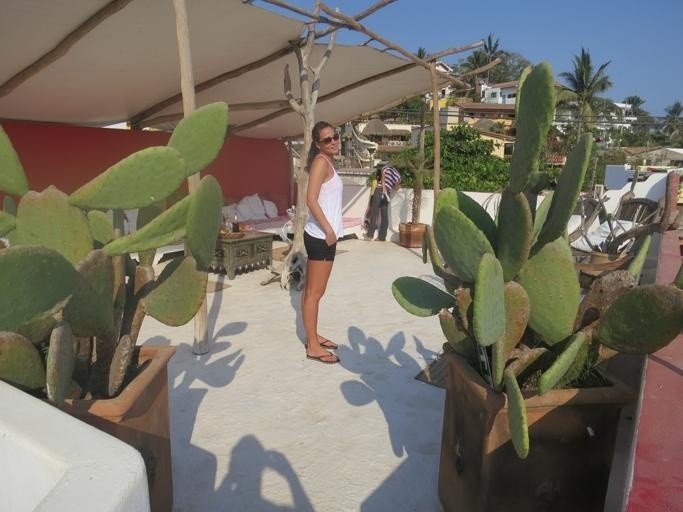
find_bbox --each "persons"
[362,169,380,229]
[297,121,342,364]
[361,164,402,243]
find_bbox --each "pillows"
[222,193,278,222]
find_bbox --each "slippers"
[306,339,339,349]
[306,351,341,364]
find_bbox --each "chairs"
[573,198,659,224]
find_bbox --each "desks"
[211,230,273,280]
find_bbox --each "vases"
[589,250,628,265]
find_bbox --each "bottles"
[222,211,239,232]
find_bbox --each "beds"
[222,191,364,240]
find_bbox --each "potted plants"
[391,61,683,512]
[383,145,443,248]
[0,101,229,512]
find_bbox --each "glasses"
[318,133,340,143]
